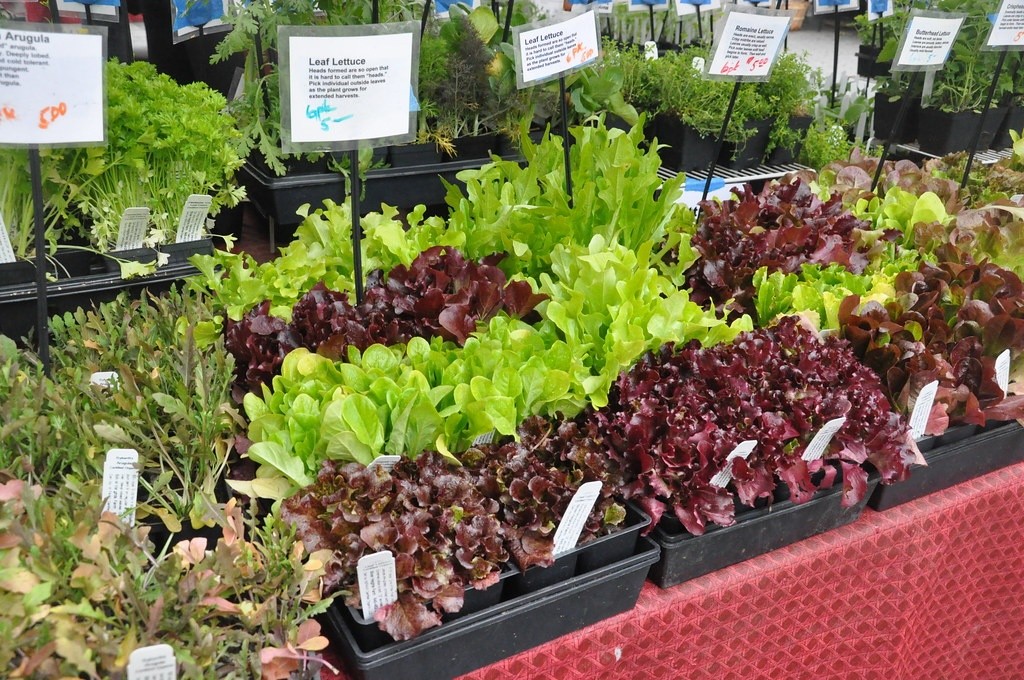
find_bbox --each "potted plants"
[0,0,1024,680]
